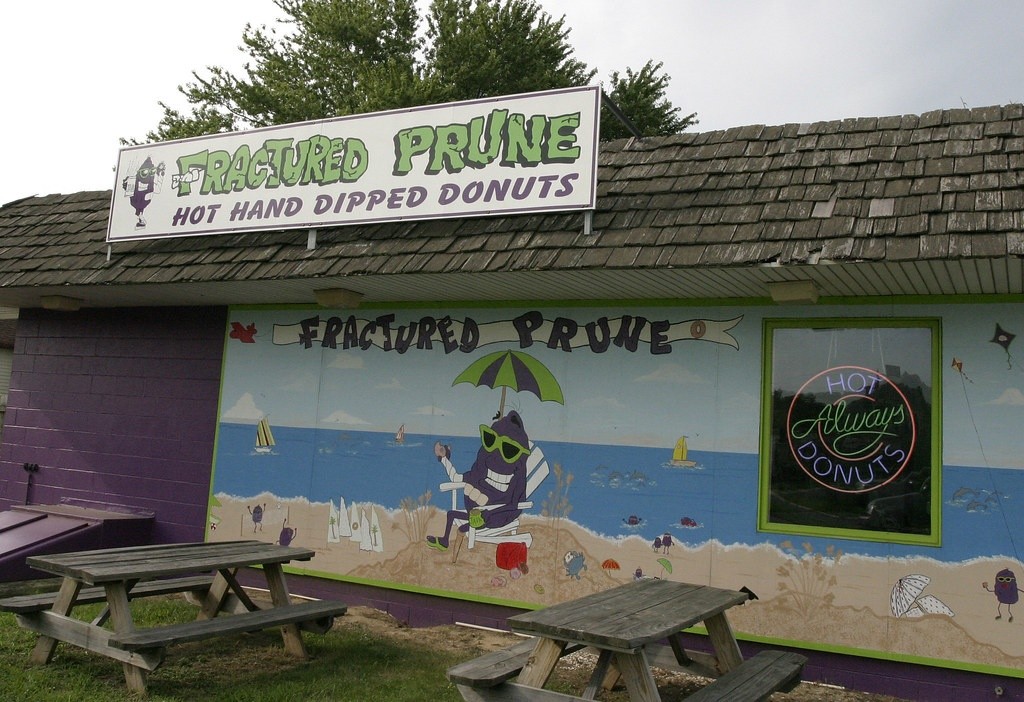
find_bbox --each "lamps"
[313,287,363,309]
[40,295,85,312]
[765,279,822,305]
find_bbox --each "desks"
[25,539,315,697]
[503,577,749,702]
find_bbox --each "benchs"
[683,649,809,702]
[107,599,348,653]
[0,575,214,614]
[448,638,586,687]
